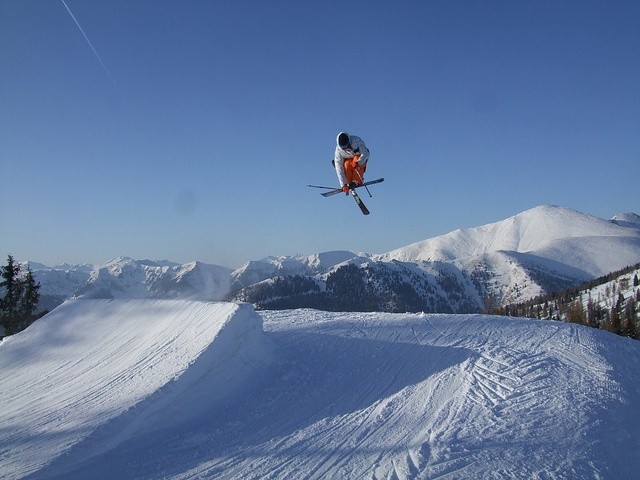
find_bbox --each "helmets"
[336,131,351,149]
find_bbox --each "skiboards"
[320,158,384,215]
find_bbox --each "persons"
[335,132,370,194]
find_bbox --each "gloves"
[342,186,349,192]
[351,161,358,168]
